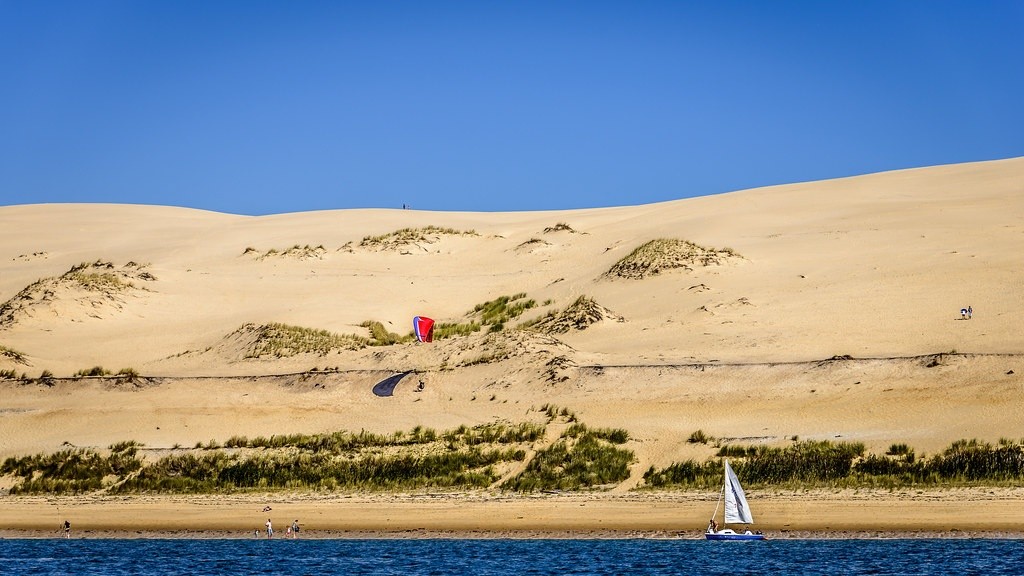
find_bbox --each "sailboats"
[704,459,767,541]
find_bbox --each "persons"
[968,305,973,319]
[415,379,423,392]
[960,308,968,319]
[285,525,292,538]
[709,520,719,533]
[265,519,273,539]
[292,519,299,537]
[254,528,259,538]
[745,526,750,533]
[755,529,762,535]
[63,521,71,539]
